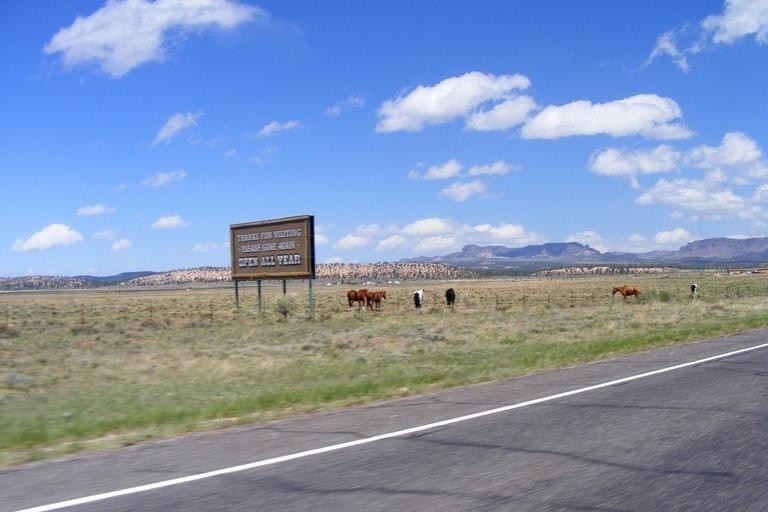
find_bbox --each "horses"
[445,287,456,306]
[612,285,641,301]
[690,283,698,299]
[347,289,368,307]
[413,288,426,308]
[366,291,387,310]
